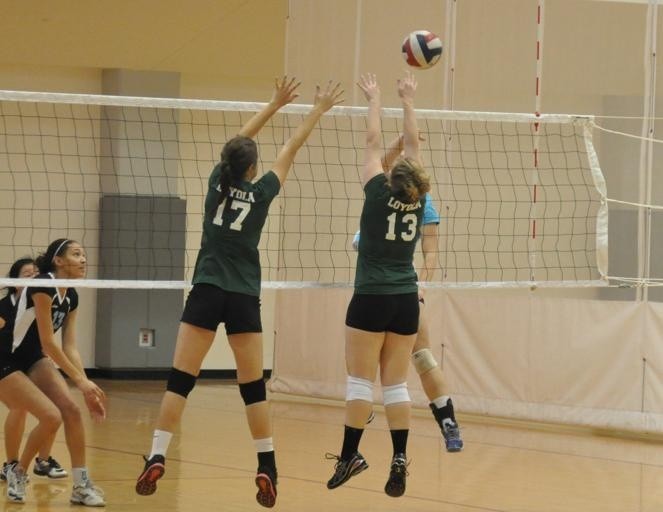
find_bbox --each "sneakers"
[256,465,278,508]
[440,425,464,454]
[5,462,27,504]
[385,450,408,498]
[1,461,30,483]
[325,451,368,490]
[69,484,107,508]
[135,455,166,496]
[35,455,69,479]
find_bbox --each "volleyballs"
[400,30,443,69]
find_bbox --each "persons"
[0,239,111,506]
[135,73,346,507]
[353,128,463,452]
[0,258,69,484]
[325,72,430,498]
